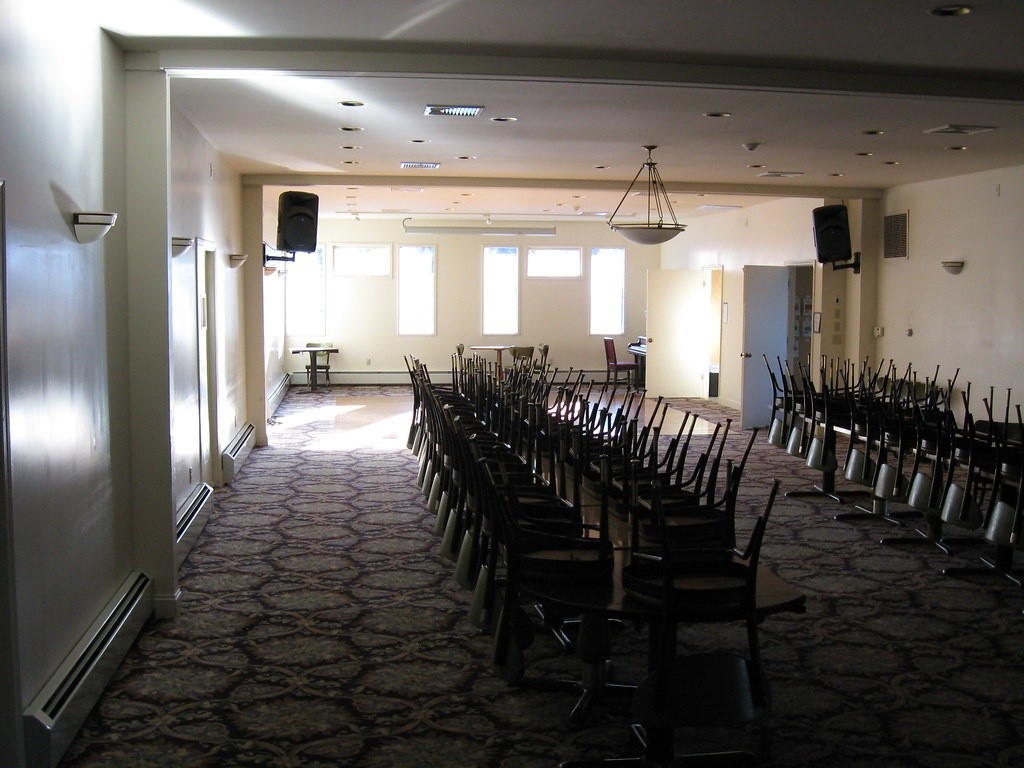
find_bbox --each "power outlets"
[189,467,193,480]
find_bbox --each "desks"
[833,434,926,529]
[878,446,987,558]
[470,345,514,381]
[497,499,708,725]
[939,469,1024,590]
[783,413,873,506]
[538,469,601,507]
[520,546,806,768]
[291,348,338,395]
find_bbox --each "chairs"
[504,347,535,374]
[401,350,1024,713]
[305,343,333,392]
[603,337,640,395]
[456,343,483,375]
[533,343,549,374]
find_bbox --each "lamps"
[171,236,195,258]
[71,210,118,245]
[228,253,248,269]
[941,260,965,275]
[606,145,688,246]
[264,266,277,277]
[401,217,560,236]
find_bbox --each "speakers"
[812,205,852,264]
[276,191,318,253]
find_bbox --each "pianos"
[626,334,646,394]
